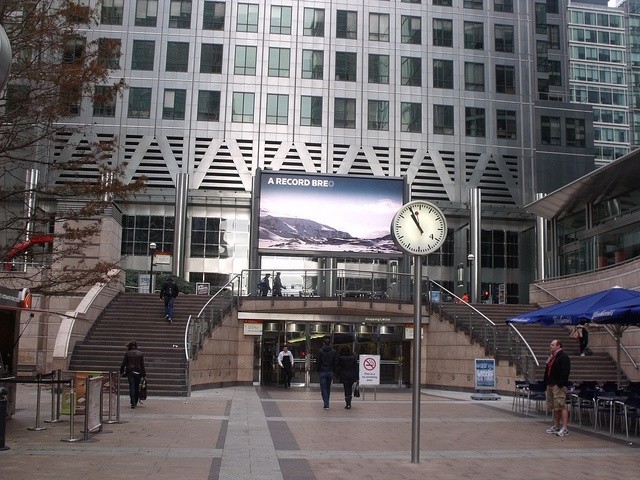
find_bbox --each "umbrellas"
[505,284,640,389]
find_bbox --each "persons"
[316,338,336,409]
[160,278,179,323]
[337,345,358,408]
[273,272,286,297]
[119,340,146,409]
[260,274,271,296]
[575,323,589,357]
[462,293,469,303]
[543,339,571,437]
[277,346,294,390]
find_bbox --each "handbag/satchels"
[139,379,147,400]
[570,329,578,340]
[577,328,583,337]
[583,347,592,356]
[354,383,360,397]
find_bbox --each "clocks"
[390,199,448,257]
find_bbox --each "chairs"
[429,380,510,480]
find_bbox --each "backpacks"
[166,282,176,295]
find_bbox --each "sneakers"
[165,314,169,318]
[323,407,329,409]
[546,426,561,433]
[556,427,569,437]
[168,318,171,321]
[580,354,585,356]
[131,404,135,407]
[345,404,351,409]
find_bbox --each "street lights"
[147,241,157,295]
[466,253,475,303]
